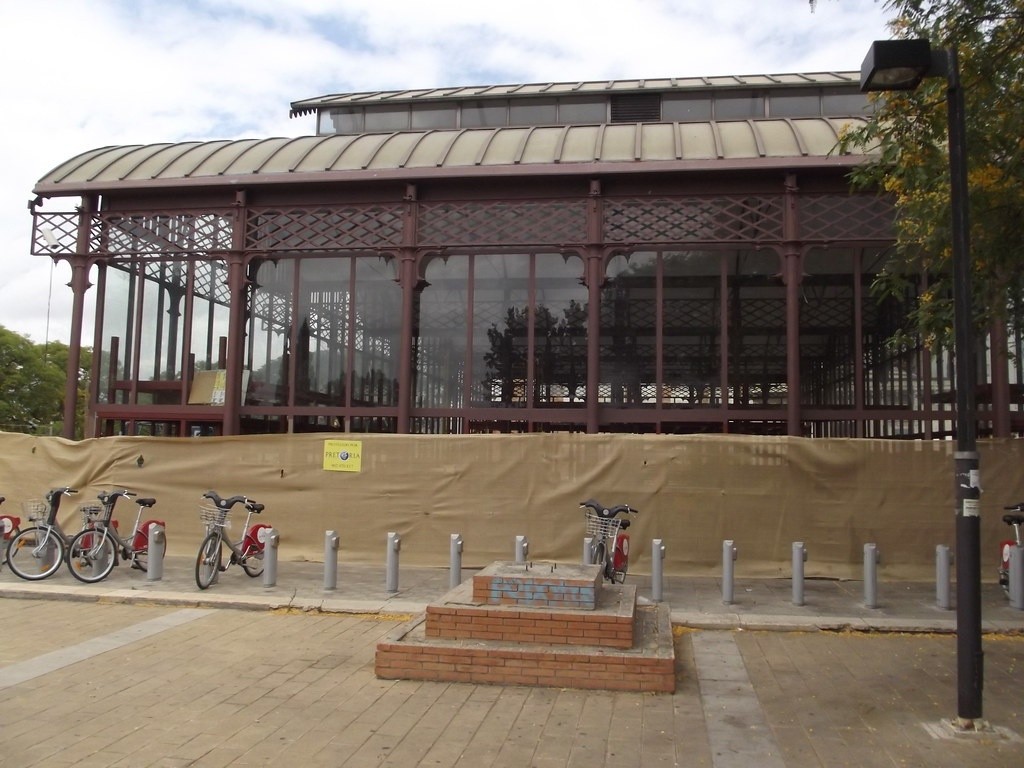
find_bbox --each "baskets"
[22,498,49,521]
[199,503,231,527]
[586,513,621,538]
[80,503,113,522]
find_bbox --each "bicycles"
[5,486,120,580]
[578,499,639,585]
[194,490,273,590]
[996,501,1024,605]
[65,487,168,585]
[0,496,21,570]
